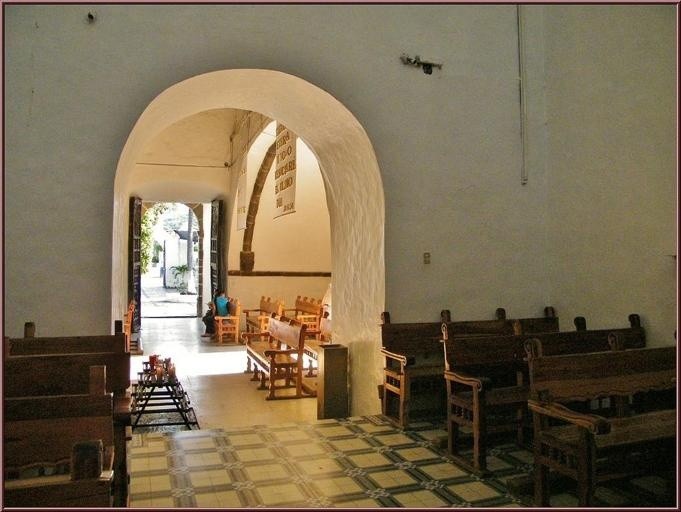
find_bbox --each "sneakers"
[201,332,217,339]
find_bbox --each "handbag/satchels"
[216,297,228,316]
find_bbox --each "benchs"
[214,296,332,401]
[4,320,131,508]
[378,307,678,507]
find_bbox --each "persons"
[200,289,223,338]
[215,290,231,317]
